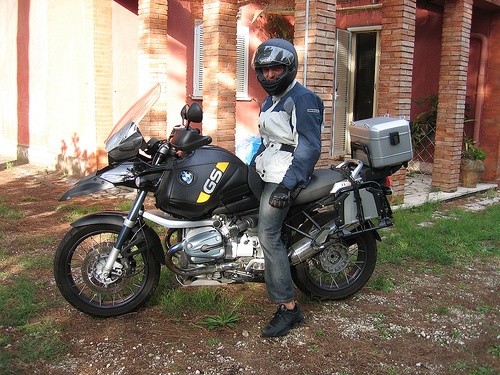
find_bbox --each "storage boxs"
[348,114,414,168]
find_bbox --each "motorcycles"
[53,82,414,319]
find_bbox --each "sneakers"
[237,275,265,283]
[260,302,302,336]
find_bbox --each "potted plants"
[461,144,486,188]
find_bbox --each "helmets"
[253,39,298,95]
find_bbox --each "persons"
[248,38,324,337]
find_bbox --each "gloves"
[269,183,291,208]
